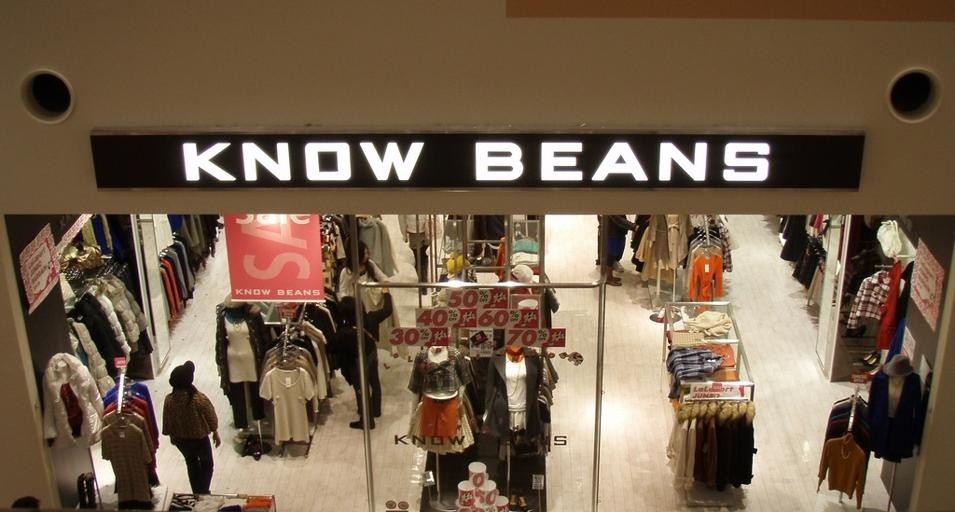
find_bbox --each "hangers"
[111,378,135,429]
[696,228,719,259]
[275,332,299,375]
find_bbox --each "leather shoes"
[861,351,880,367]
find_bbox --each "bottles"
[454,463,509,512]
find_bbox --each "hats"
[169,361,195,388]
[511,263,536,295]
[881,352,914,377]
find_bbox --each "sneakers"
[349,404,382,430]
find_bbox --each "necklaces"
[892,377,901,389]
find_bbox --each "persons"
[482,343,544,437]
[338,240,387,310]
[866,354,921,464]
[161,360,221,494]
[330,287,392,428]
[607,215,639,286]
[613,260,624,273]
[406,344,475,438]
[398,213,430,295]
